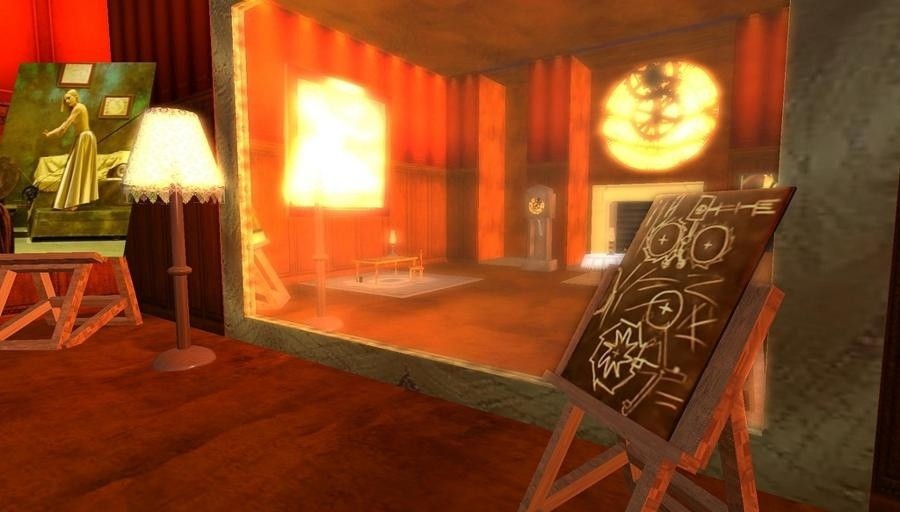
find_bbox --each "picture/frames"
[97,94,135,121]
[57,62,97,90]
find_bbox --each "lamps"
[118,104,224,372]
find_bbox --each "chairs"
[408,248,426,283]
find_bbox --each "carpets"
[303,268,486,300]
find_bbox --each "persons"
[42,89,99,211]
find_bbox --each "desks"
[354,256,420,287]
